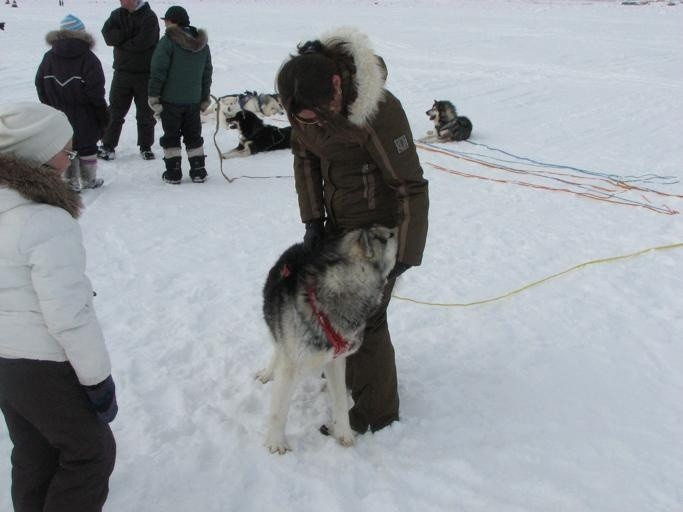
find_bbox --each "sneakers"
[139,146,155,160]
[96,145,115,159]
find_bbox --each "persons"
[98,0,160,160]
[34,13,110,193]
[0,99,119,512]
[273,25,429,436]
[148,6,213,183]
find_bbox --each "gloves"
[302,218,326,260]
[85,371,119,423]
[148,95,163,114]
[199,97,211,111]
[385,258,414,284]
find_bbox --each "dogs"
[419,99,472,145]
[253,221,402,457]
[221,107,293,160]
[203,90,285,117]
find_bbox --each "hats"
[0,99,74,172]
[59,14,87,38]
[161,5,192,28]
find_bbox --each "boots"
[161,148,183,184]
[62,153,81,194]
[80,154,104,190]
[186,146,208,184]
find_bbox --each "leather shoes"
[320,405,400,439]
